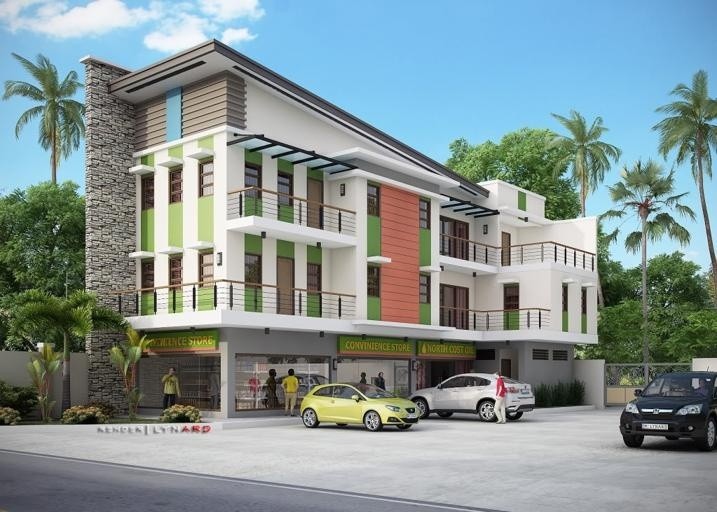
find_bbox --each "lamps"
[339,184,346,196]
[482,224,488,234]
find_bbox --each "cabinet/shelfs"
[178,356,221,410]
[234,361,320,409]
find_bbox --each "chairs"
[665,379,686,396]
